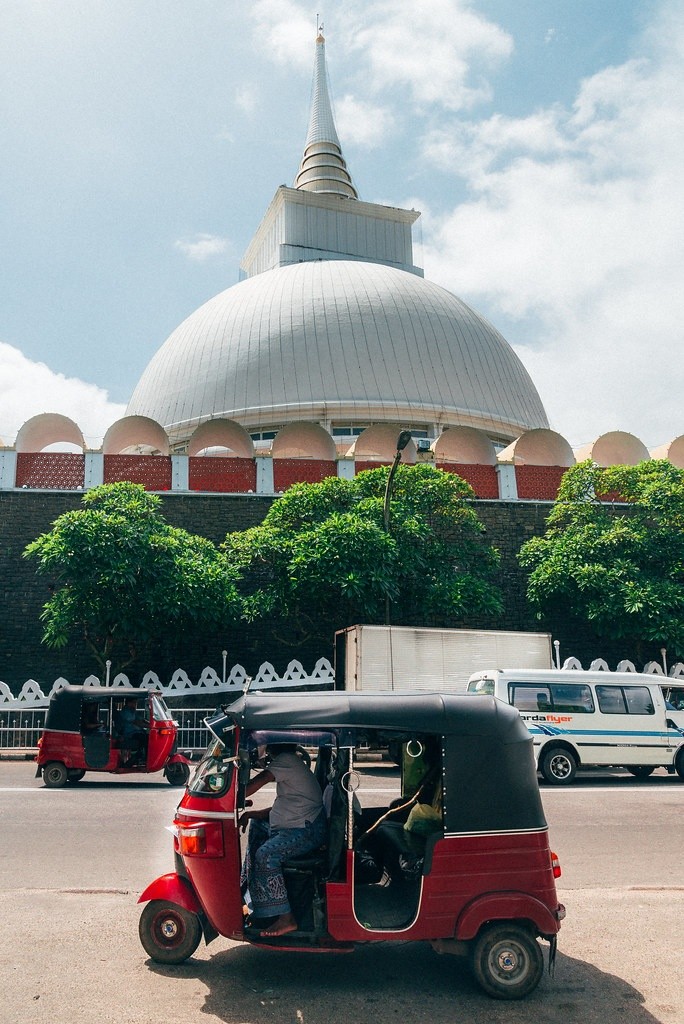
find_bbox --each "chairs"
[112,702,144,750]
[536,693,552,711]
[81,703,110,768]
[374,774,443,884]
[281,815,346,919]
[601,697,619,713]
[632,693,651,713]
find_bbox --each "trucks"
[333,624,552,763]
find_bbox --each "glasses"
[130,700,138,704]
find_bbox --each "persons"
[661,687,683,710]
[361,734,446,889]
[114,700,155,771]
[242,742,331,935]
[82,703,105,736]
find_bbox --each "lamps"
[417,440,430,452]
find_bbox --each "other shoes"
[132,758,147,767]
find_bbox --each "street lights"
[384,429,414,624]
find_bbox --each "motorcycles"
[35,685,193,789]
[135,692,569,1002]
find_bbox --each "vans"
[465,667,684,781]
